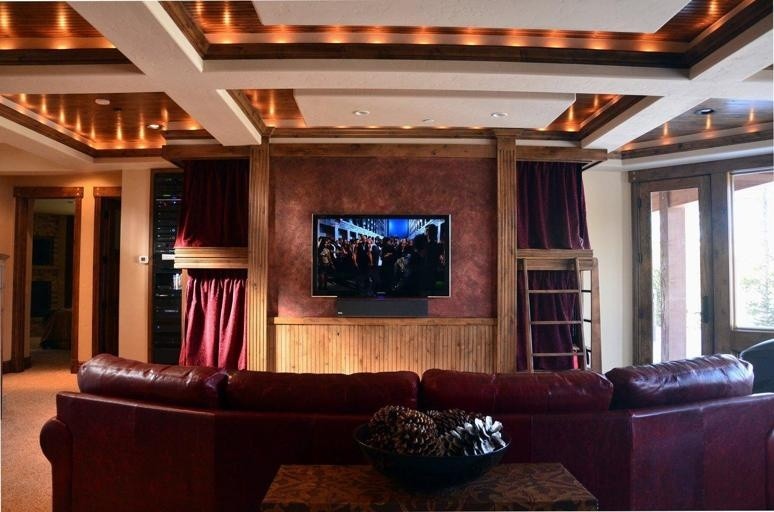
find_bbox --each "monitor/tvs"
[311,214,452,298]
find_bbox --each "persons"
[316,223,448,295]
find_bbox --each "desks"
[261,460,597,508]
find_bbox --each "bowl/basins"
[352,419,514,490]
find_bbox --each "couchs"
[38,336,773,509]
